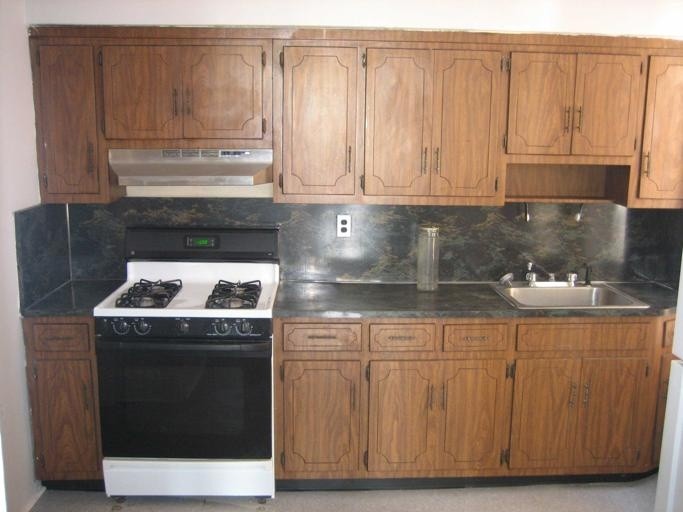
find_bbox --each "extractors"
[105,146,272,187]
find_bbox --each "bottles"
[415,226,440,291]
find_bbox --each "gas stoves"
[93,257,279,338]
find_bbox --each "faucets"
[547,273,555,282]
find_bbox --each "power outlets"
[336,214,351,240]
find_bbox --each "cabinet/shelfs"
[510,317,658,477]
[358,41,510,207]
[99,38,271,150]
[506,45,644,166]
[273,316,362,481]
[362,316,509,479]
[272,38,358,208]
[612,48,682,210]
[29,36,126,204]
[654,314,676,469]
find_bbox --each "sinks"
[489,280,651,310]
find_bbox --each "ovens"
[96,343,277,506]
